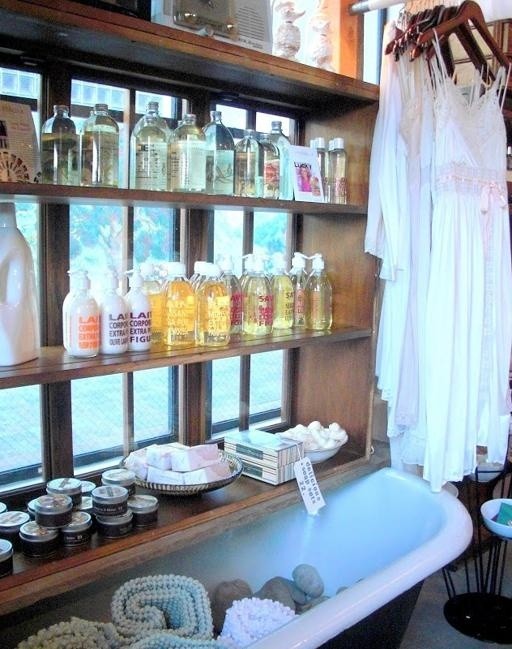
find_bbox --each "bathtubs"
[0,466,474,648]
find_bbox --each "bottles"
[129,102,349,205]
[143,261,231,347]
[38,101,118,189]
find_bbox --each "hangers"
[384,0,511,87]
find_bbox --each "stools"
[443,458,512,644]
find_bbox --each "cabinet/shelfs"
[1,0,416,615]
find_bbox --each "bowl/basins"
[275,428,349,464]
[480,498,512,540]
